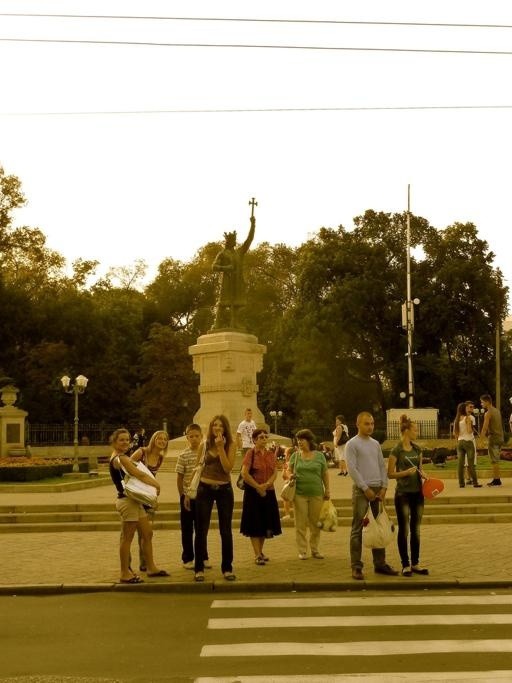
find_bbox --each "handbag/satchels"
[237,470,255,490]
[337,431,349,445]
[186,464,205,499]
[362,500,395,548]
[280,478,296,502]
[120,460,158,509]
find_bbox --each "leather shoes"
[224,572,235,580]
[352,568,364,579]
[413,566,428,574]
[403,568,412,576]
[375,564,398,575]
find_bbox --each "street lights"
[60,374,90,473]
[473,407,487,437]
[270,410,283,434]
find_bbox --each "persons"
[280,447,298,527]
[465,401,481,485]
[452,403,483,488]
[509,397,512,435]
[175,424,212,569]
[137,429,146,447]
[108,430,169,582]
[236,409,257,455]
[212,216,256,328]
[479,394,504,486]
[345,411,399,580]
[184,414,238,581]
[332,415,349,475]
[240,429,282,564]
[387,414,429,576]
[130,430,168,573]
[286,429,330,559]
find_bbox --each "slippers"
[120,576,143,583]
[147,570,169,576]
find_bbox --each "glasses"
[259,436,268,439]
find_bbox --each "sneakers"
[194,572,205,581]
[140,566,147,570]
[204,561,212,569]
[183,562,194,569]
[466,481,472,484]
[460,485,465,488]
[312,552,323,559]
[338,471,344,475]
[283,515,291,518]
[255,557,265,565]
[260,553,268,560]
[473,484,482,487]
[343,471,348,476]
[487,479,501,487]
[299,553,307,559]
